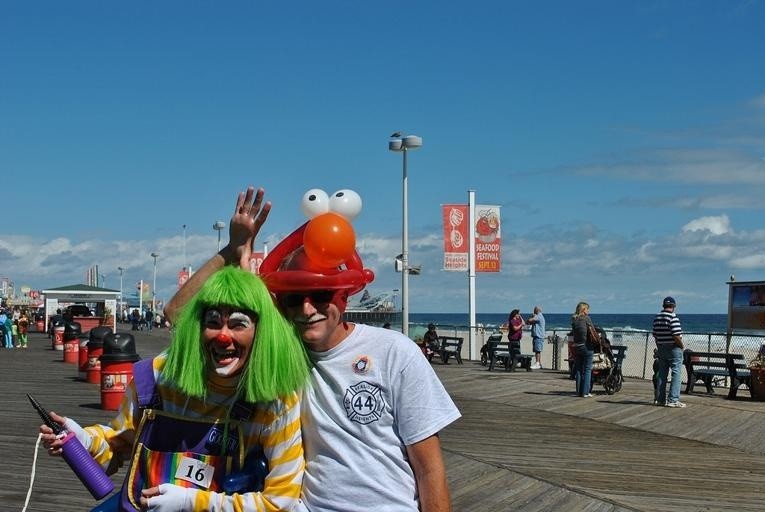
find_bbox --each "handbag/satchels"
[585,316,601,348]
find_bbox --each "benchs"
[432,336,464,364]
[565,345,628,383]
[683,351,751,399]
[487,340,534,372]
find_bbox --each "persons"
[527,305,546,369]
[570,302,596,398]
[129,308,154,332]
[423,323,450,364]
[508,310,525,366]
[39,266,308,512]
[161,186,462,512]
[0,299,44,350]
[653,296,687,408]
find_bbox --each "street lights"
[213,222,225,253]
[151,253,159,311]
[101,274,106,288]
[118,267,123,320]
[389,135,422,337]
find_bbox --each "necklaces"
[208,376,237,389]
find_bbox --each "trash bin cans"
[63,322,81,364]
[98,332,142,411]
[52,318,66,351]
[86,327,113,384]
[35,314,45,332]
[78,331,90,372]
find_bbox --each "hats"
[663,296,676,308]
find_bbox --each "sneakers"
[530,364,542,369]
[654,399,665,406]
[665,399,687,408]
[583,393,593,398]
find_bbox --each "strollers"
[480,331,503,365]
[589,328,621,395]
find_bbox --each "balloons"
[257,188,374,296]
